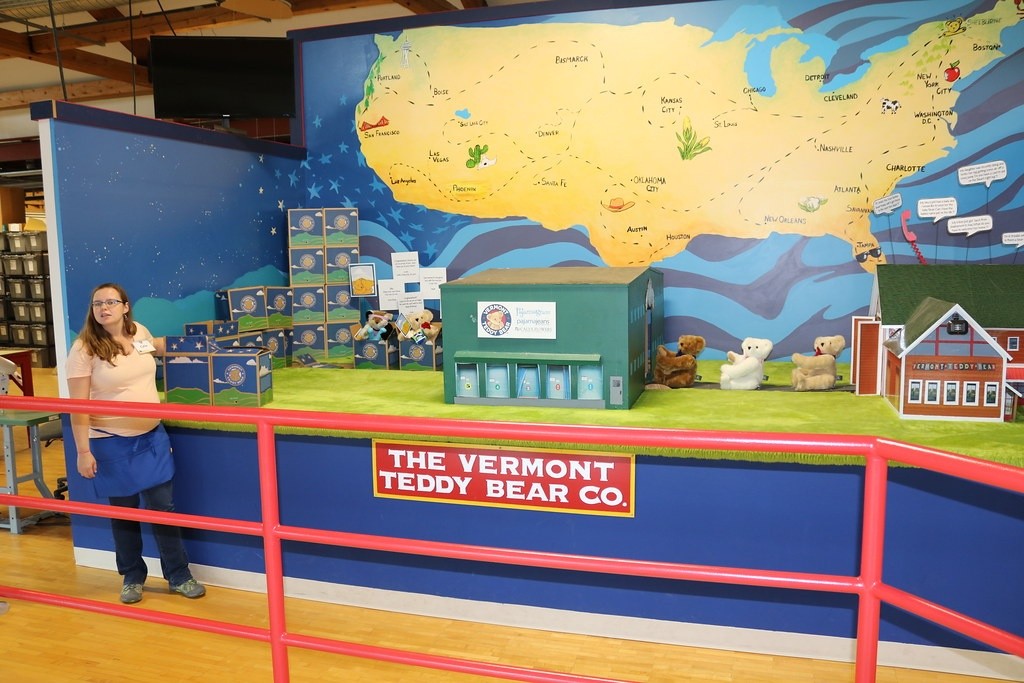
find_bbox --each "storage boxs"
[153,208,444,406]
[0,230,55,368]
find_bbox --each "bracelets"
[78,450,91,454]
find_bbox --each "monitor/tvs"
[148,33,296,120]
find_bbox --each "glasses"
[92,299,123,307]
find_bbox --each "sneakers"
[169,578,206,597]
[121,584,142,603]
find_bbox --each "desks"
[0,411,61,533]
[0,350,36,397]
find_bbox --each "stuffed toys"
[653,335,705,388]
[363,309,438,341]
[720,337,773,389]
[792,335,846,391]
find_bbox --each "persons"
[66,283,206,603]
